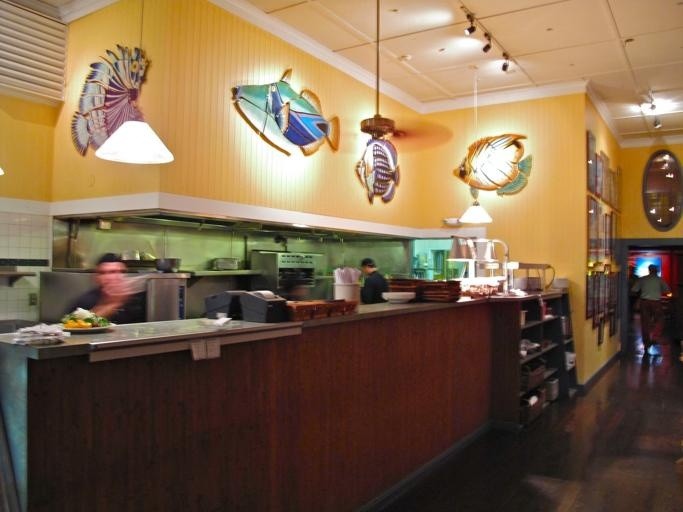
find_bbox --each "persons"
[359,259,389,304]
[628,265,670,351]
[61,254,147,323]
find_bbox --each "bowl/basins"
[154,258,181,273]
[382,291,416,305]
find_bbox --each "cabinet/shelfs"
[488,285,579,435]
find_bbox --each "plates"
[58,321,117,335]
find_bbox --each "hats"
[360,258,373,266]
[97,252,121,263]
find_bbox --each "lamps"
[464,12,509,73]
